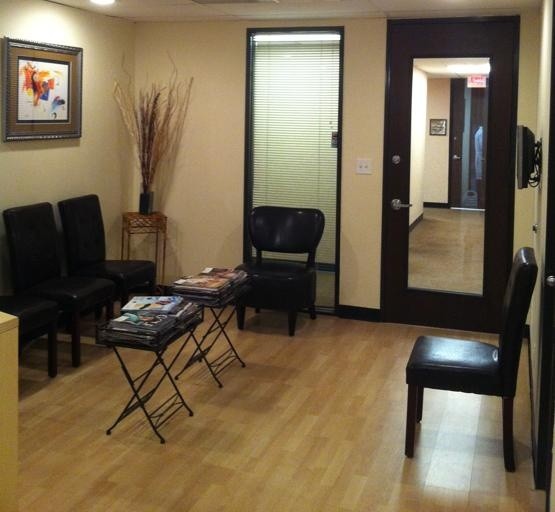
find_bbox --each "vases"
[139,190,154,214]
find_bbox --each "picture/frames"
[430,118,447,136]
[1,35,83,142]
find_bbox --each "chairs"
[405,247,538,473]
[1,194,156,378]
[233,205,325,337]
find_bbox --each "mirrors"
[406,56,492,298]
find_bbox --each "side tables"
[95,276,257,443]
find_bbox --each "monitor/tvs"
[516,125,535,190]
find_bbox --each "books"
[96,266,250,350]
